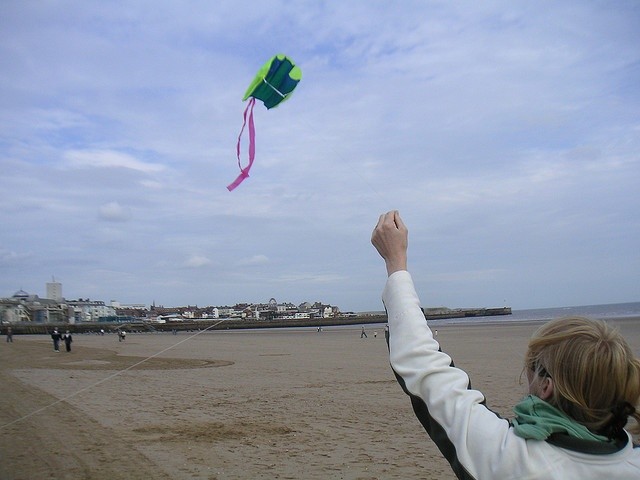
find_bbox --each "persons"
[359,325,367,338]
[5,326,13,342]
[49,326,62,350]
[382,325,389,336]
[316,326,320,332]
[118,326,127,342]
[59,328,74,354]
[434,328,438,337]
[370,209,639,480]
[372,330,378,338]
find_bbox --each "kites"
[227,56,302,191]
